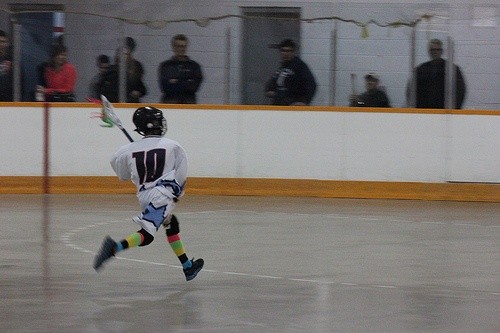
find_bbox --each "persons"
[0,29,14,103]
[404,38,467,110]
[263,37,318,104]
[35,43,78,101]
[347,72,392,108]
[158,33,203,105]
[93,106,204,282]
[92,37,147,101]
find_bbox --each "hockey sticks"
[89,94,134,144]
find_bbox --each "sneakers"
[184,258,204,281]
[93,236,117,270]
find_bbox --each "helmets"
[132,106,162,129]
